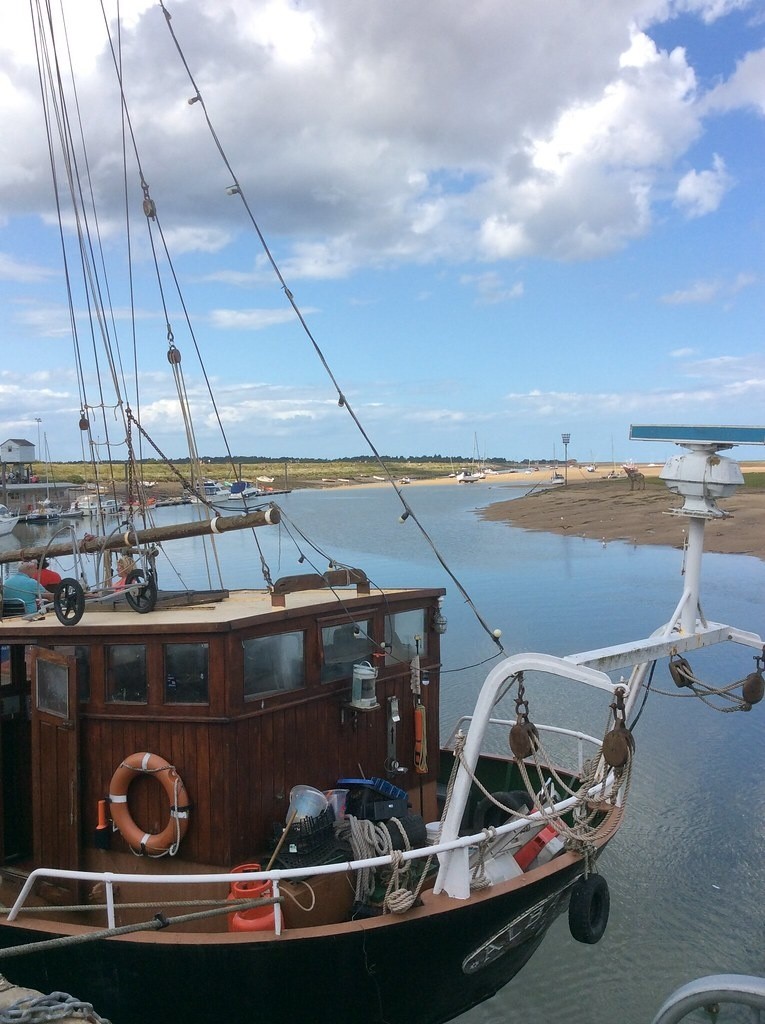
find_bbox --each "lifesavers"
[108,751,190,855]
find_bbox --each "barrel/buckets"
[285,785,328,824]
[423,822,441,866]
[322,788,351,822]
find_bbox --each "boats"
[58,506,83,518]
[0,503,20,536]
[120,495,156,511]
[154,475,292,509]
[70,495,123,516]
[320,475,410,485]
[26,506,61,522]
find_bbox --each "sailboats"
[444,431,622,485]
[0,0,765,1024]
